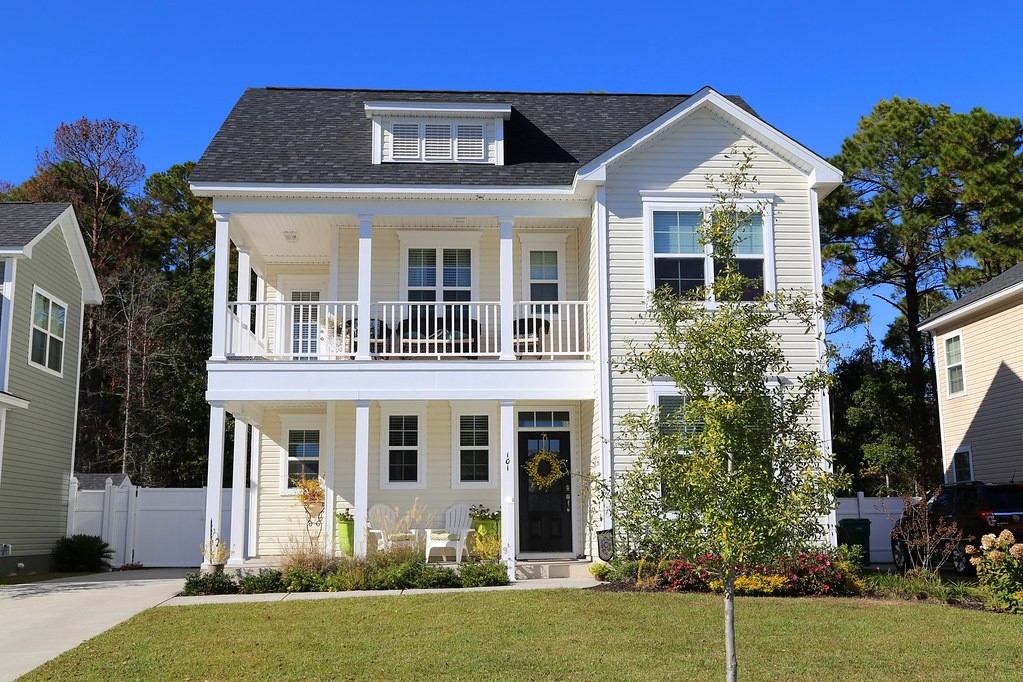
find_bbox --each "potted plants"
[200,534,233,578]
[319,314,341,338]
[289,473,329,519]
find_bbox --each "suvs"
[890,474,1023,579]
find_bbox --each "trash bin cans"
[839,518,872,567]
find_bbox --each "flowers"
[469,504,499,519]
[333,508,354,521]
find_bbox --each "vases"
[337,521,355,557]
[475,519,498,537]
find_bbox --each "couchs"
[344,317,393,360]
[513,317,550,360]
[395,317,481,360]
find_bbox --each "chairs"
[367,502,419,554]
[425,503,477,563]
